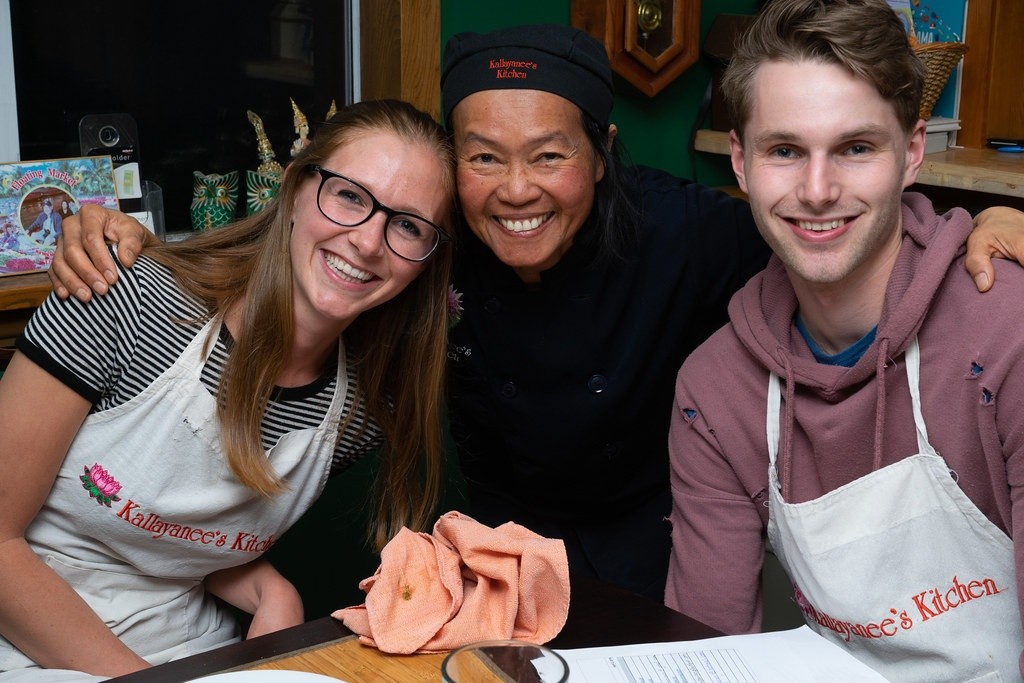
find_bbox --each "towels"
[330,510,573,656]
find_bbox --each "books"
[910,0,968,157]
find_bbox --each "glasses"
[304,164,452,262]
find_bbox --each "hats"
[440,25,614,123]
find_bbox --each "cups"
[439,638,572,683]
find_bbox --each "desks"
[86,571,885,683]
[2,239,191,373]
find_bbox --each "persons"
[46,21,1024,610]
[666,0,1024,683]
[56,200,74,219]
[25,200,63,246]
[288,97,311,157]
[1,94,465,683]
